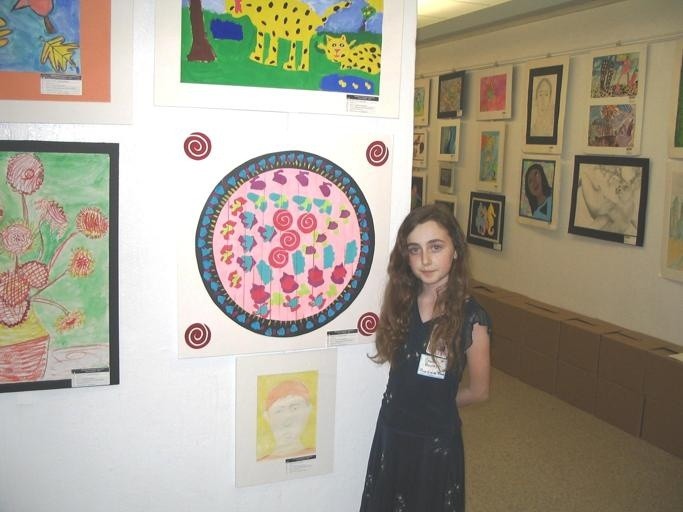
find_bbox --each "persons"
[533,76,554,136]
[356,204,496,511]
[521,165,552,221]
[255,378,316,462]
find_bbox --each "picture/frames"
[660,162,682,284]
[467,191,506,252]
[433,193,457,218]
[438,118,461,162]
[565,152,650,248]
[520,53,571,154]
[412,172,427,209]
[414,130,429,169]
[514,154,562,232]
[470,120,507,192]
[582,41,651,156]
[475,63,514,120]
[437,162,455,194]
[437,69,465,118]
[668,43,683,160]
[414,78,431,127]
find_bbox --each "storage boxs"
[461,277,682,462]
